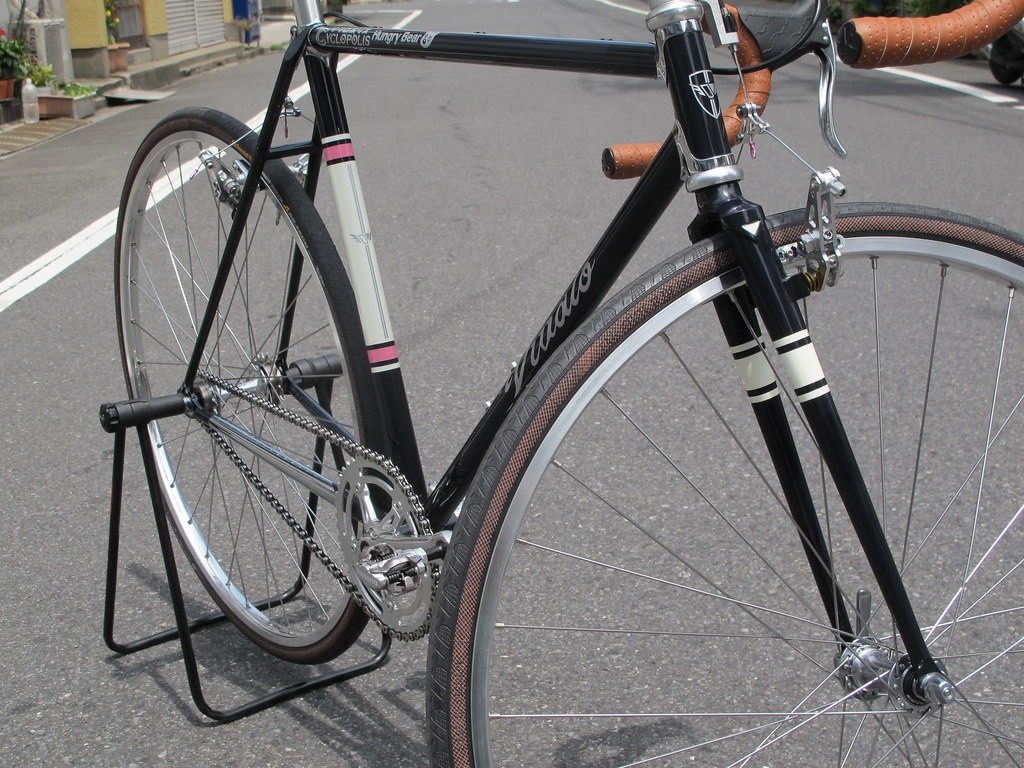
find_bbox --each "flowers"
[104,1,122,44]
[0,28,7,37]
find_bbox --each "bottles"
[21,78,40,123]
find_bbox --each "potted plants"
[28,63,99,120]
[0,37,32,99]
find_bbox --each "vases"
[108,41,130,72]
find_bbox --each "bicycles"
[98,0,1024,768]
